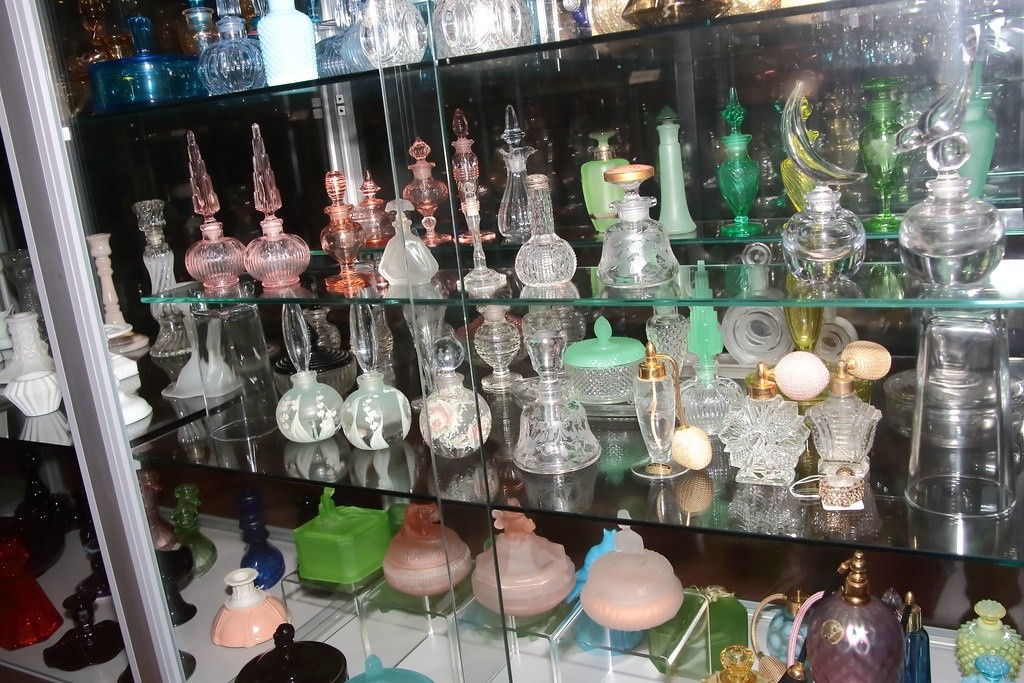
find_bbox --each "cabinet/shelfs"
[0,0,1024,683]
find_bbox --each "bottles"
[0,0,1024,683]
